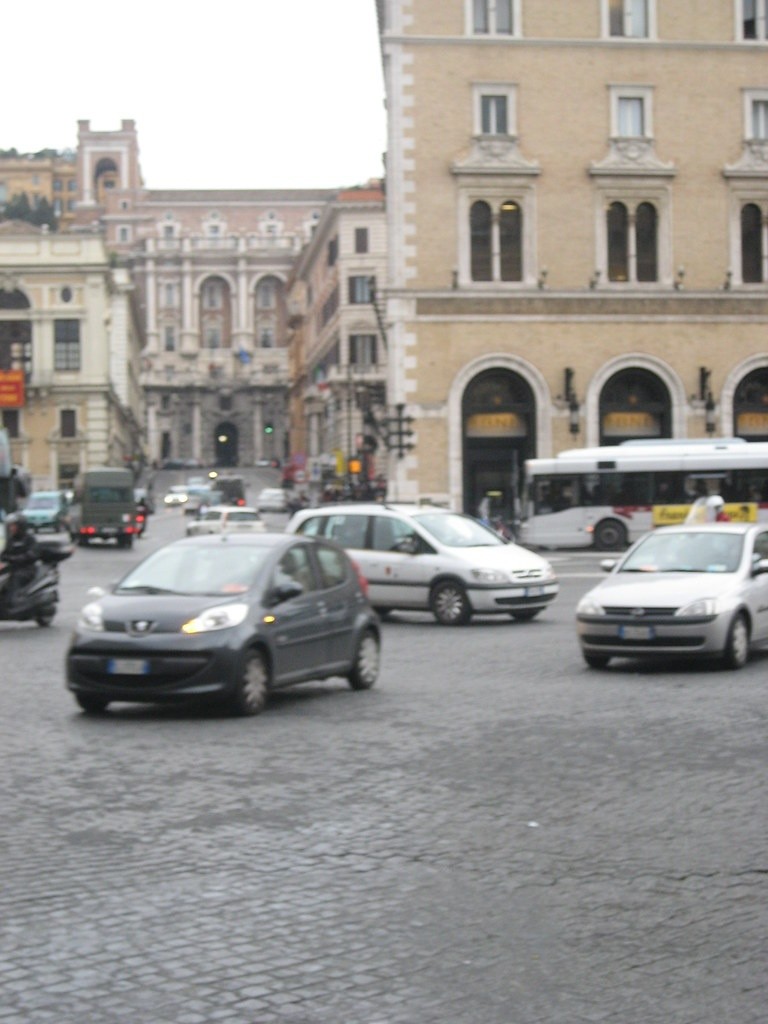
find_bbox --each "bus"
[508,436,768,551]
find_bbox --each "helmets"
[706,496,724,513]
[6,514,28,528]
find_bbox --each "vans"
[69,465,139,551]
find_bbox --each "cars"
[6,475,289,533]
[63,529,383,718]
[571,519,767,675]
[185,503,268,538]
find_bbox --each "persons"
[282,470,387,512]
[706,495,733,523]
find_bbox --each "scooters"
[1,510,74,632]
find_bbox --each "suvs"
[285,502,561,630]
